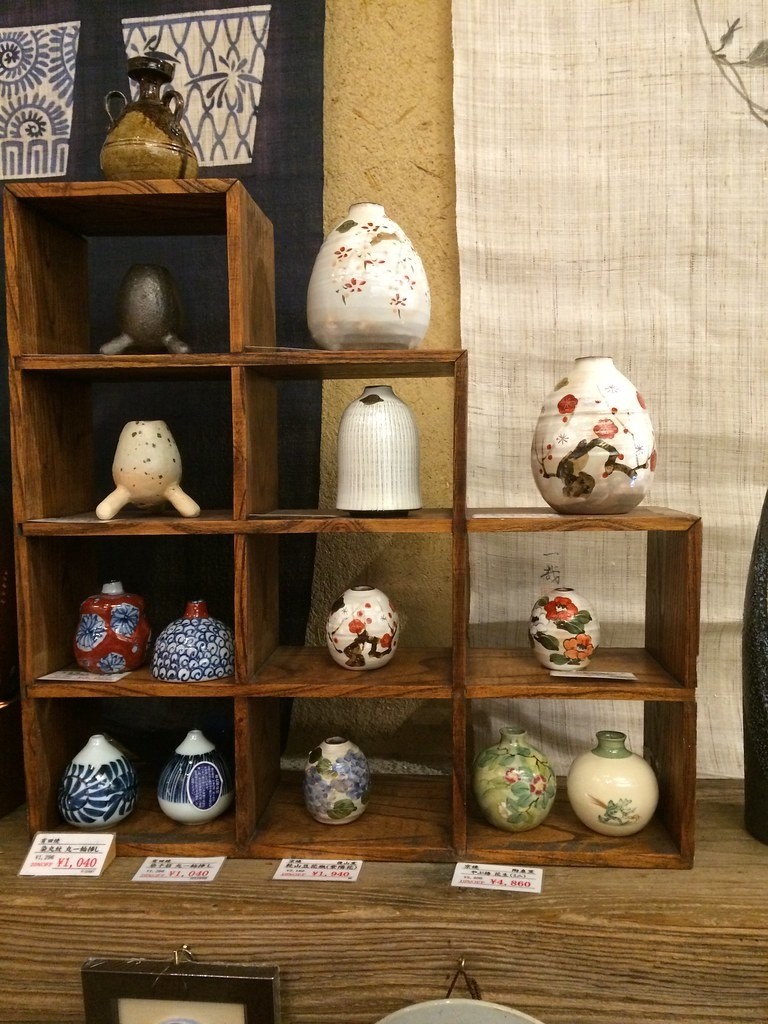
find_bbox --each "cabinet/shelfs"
[0,175,702,869]
